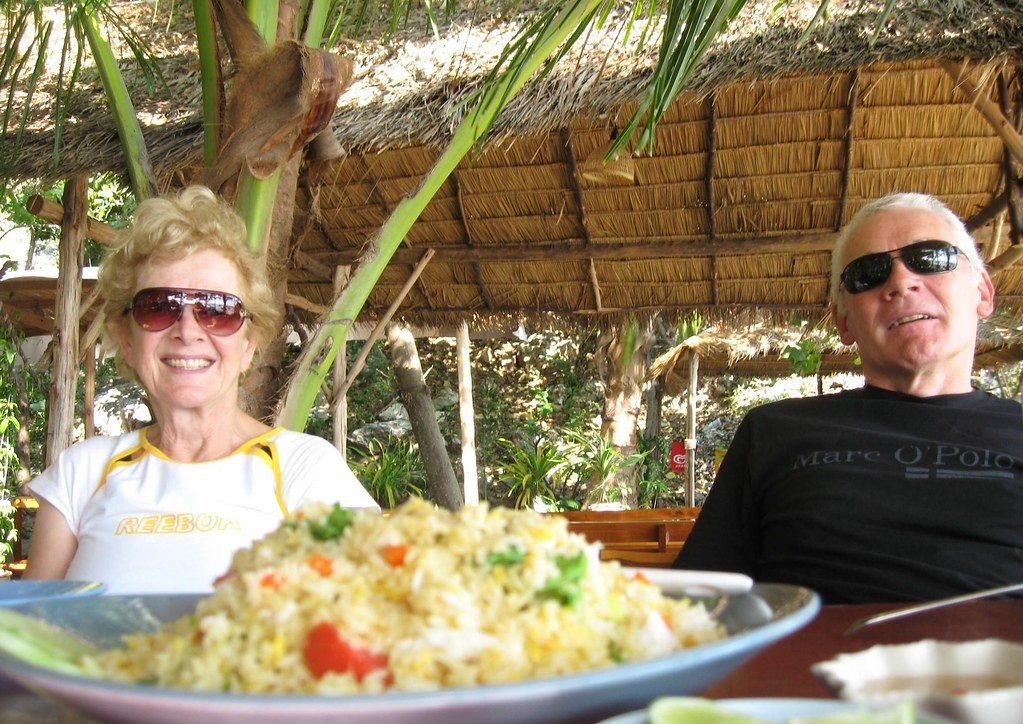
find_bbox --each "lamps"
[581,118,635,186]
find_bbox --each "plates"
[0,580,819,724]
[810,639,1023,723]
[593,699,953,723]
[0,579,107,610]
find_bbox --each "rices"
[70,495,732,698]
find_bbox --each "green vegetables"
[296,498,634,666]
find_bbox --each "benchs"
[546,508,703,568]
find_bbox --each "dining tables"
[0,603,1023,724]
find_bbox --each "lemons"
[646,694,917,724]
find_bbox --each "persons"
[17,178,384,597]
[671,192,1023,604]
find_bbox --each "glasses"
[118,287,251,336]
[838,240,970,294]
